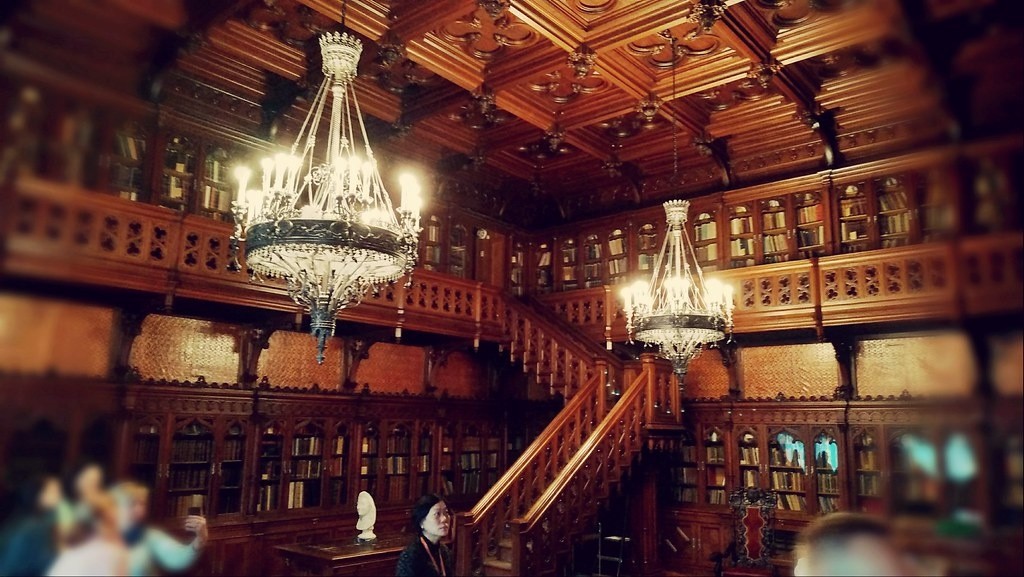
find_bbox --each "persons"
[352,490,379,542]
[394,490,459,577]
[793,510,914,577]
[0,462,209,577]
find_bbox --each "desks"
[271,530,453,577]
[765,551,795,577]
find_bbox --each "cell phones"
[188,507,200,516]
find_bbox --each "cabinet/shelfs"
[96,106,451,275]
[662,420,947,516]
[502,147,967,298]
[0,365,532,532]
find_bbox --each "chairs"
[709,486,779,577]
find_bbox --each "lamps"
[231,0,425,367]
[618,37,737,395]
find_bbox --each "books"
[673,441,887,513]
[110,124,1024,295]
[132,426,504,520]
